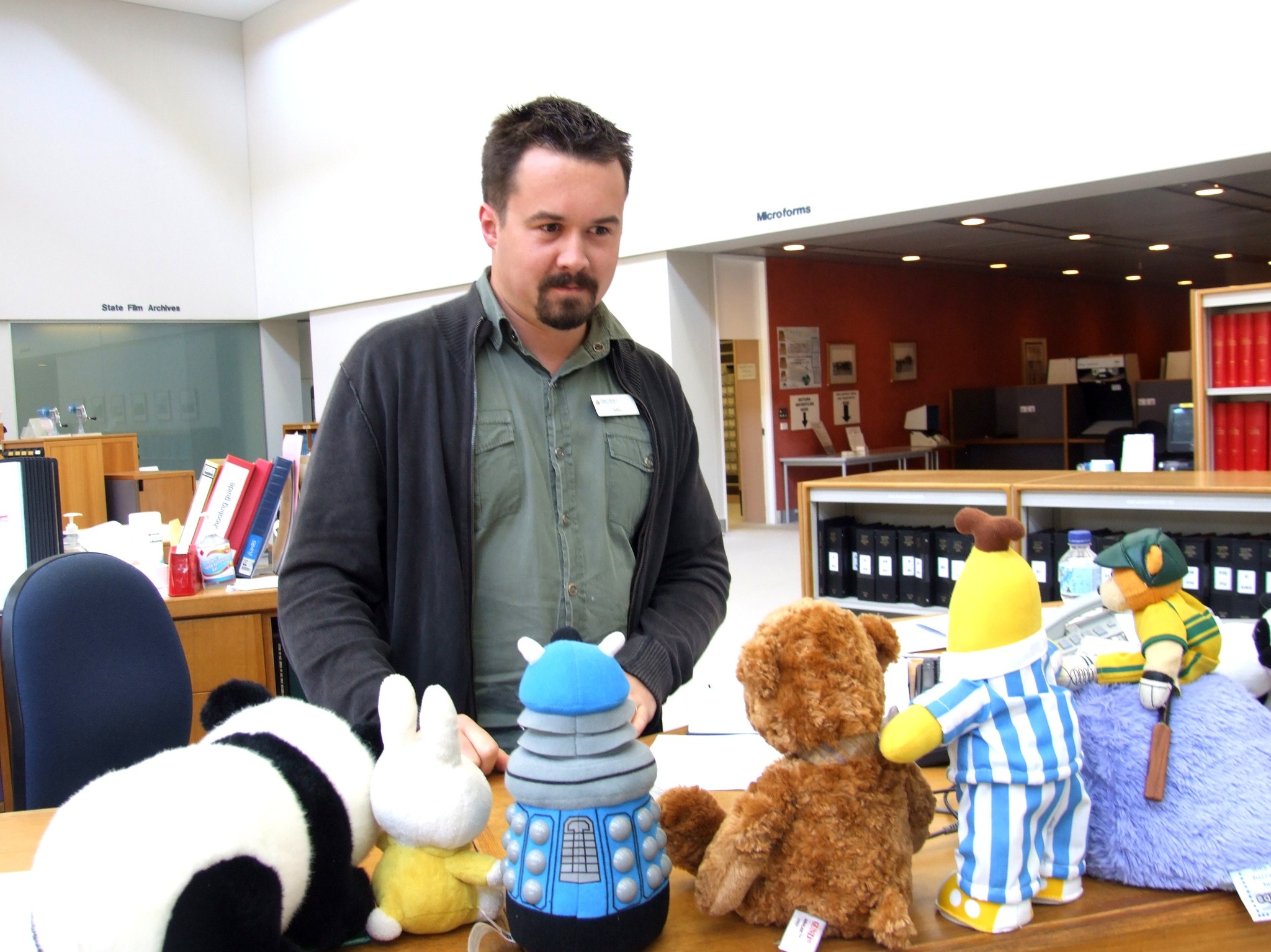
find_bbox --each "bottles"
[1057,530,1102,604]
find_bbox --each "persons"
[278,95,732,779]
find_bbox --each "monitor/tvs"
[1165,403,1193,454]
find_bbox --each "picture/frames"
[889,340,919,384]
[826,341,859,388]
[1019,337,1048,385]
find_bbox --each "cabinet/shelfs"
[797,470,1271,618]
[1190,283,1271,470]
[0,423,321,740]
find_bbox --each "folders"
[177,456,290,575]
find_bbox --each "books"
[1211,311,1271,470]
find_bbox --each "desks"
[0,727,1271,952]
[779,444,953,523]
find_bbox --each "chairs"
[0,552,192,811]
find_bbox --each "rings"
[635,706,637,712]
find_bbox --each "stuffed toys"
[501,626,673,952]
[1060,527,1221,709]
[878,507,1092,932]
[20,679,382,952]
[366,674,506,939]
[657,599,937,951]
[1065,671,1270,891]
